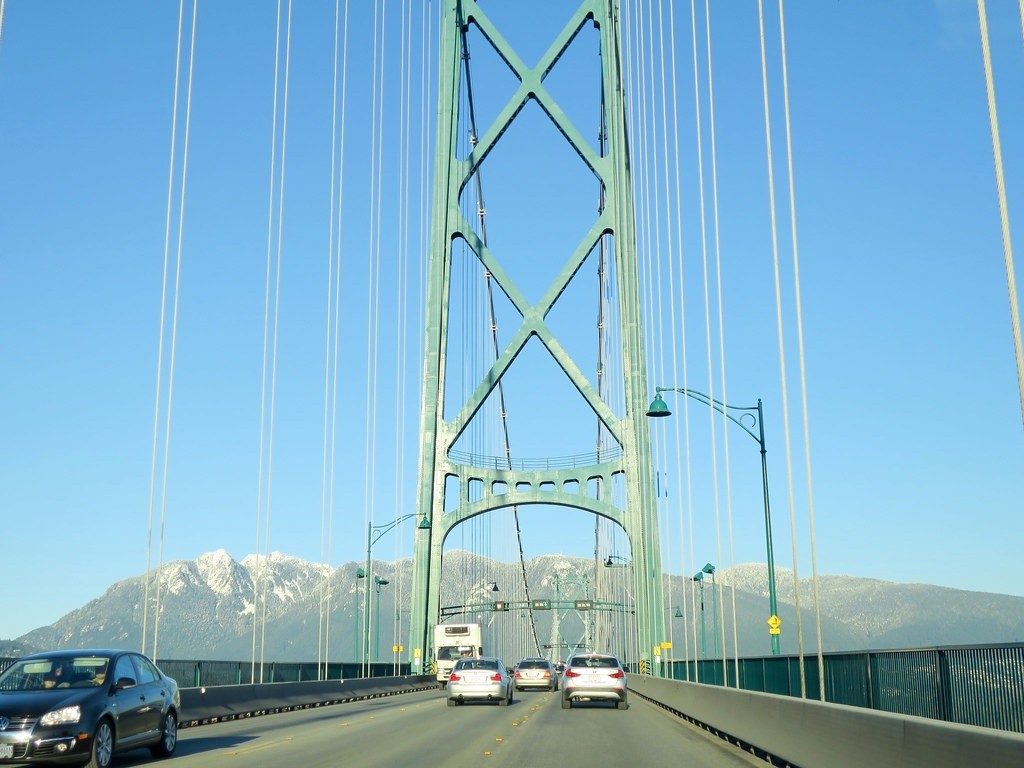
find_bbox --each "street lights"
[690,572,706,659]
[552,573,561,661]
[702,564,718,657]
[582,574,595,654]
[355,514,433,662]
[464,581,500,622]
[645,386,781,657]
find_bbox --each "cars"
[512,657,565,693]
[0,651,183,768]
[447,657,516,707]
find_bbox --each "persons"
[464,651,471,657]
[92,666,106,685]
[42,667,61,688]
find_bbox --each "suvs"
[560,652,630,710]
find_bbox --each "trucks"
[428,624,482,689]
